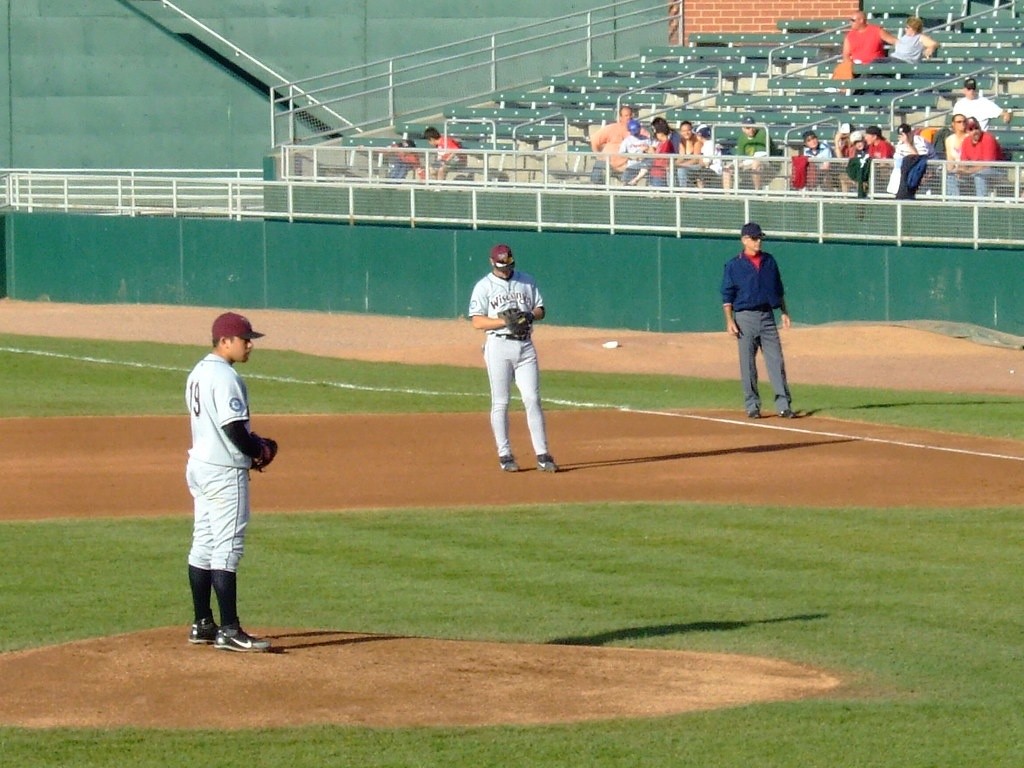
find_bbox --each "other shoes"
[749,410,761,419]
[778,409,796,418]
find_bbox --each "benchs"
[337,0,1024,199]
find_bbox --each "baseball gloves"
[252,433,277,469]
[499,308,530,335]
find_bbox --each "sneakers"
[499,455,519,471]
[538,459,559,473]
[188,609,220,644]
[213,614,272,653]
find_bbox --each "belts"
[491,331,531,341]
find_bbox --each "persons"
[589,11,1013,201]
[469,245,560,472]
[721,223,802,418]
[424,127,465,181]
[185,312,278,652]
[384,140,419,184]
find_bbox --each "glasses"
[494,263,515,270]
[953,120,965,124]
[743,234,762,241]
[966,87,976,90]
[969,126,979,131]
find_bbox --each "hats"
[866,126,882,136]
[839,123,854,134]
[741,222,765,237]
[849,130,864,145]
[964,78,976,89]
[212,312,264,342]
[897,124,911,134]
[741,117,754,124]
[965,116,979,131]
[802,130,814,143]
[627,120,640,135]
[491,244,515,268]
[691,123,710,138]
